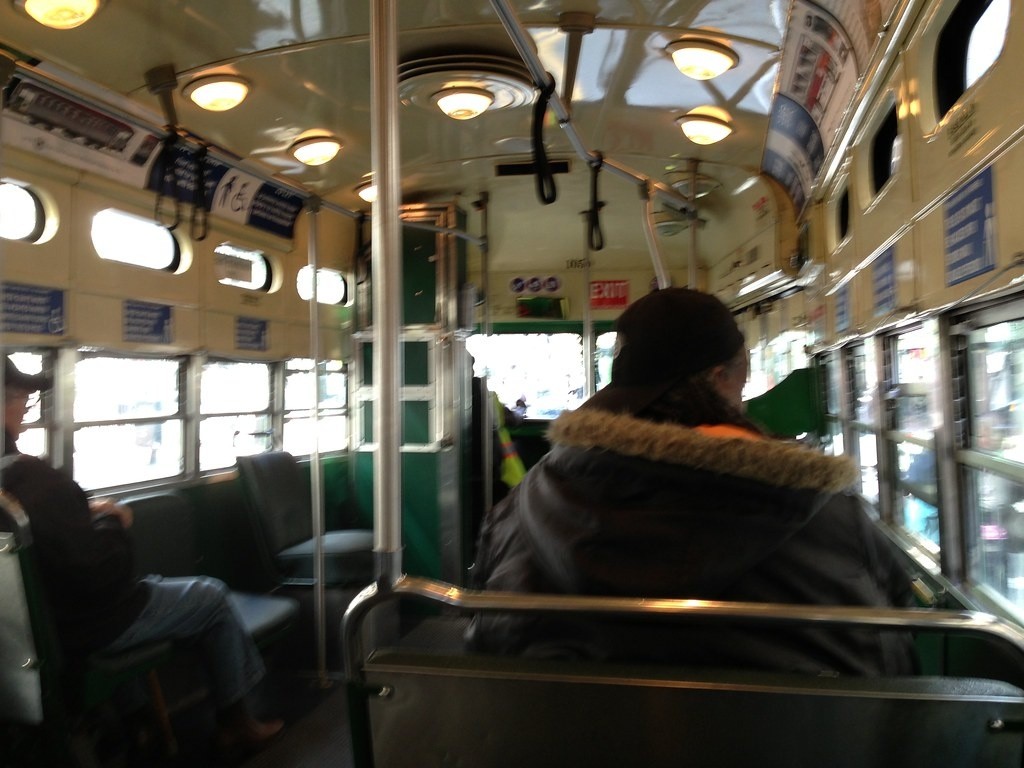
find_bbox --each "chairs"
[238,450,374,695]
[333,578,1024,768]
[114,488,306,709]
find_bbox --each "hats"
[5,355,52,394]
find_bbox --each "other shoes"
[212,713,283,753]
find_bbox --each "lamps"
[13,0,106,32]
[180,75,254,113]
[673,114,736,147]
[664,39,739,81]
[388,55,541,122]
[286,137,344,166]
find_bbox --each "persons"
[462,285,914,682]
[0,353,286,751]
[469,353,527,500]
